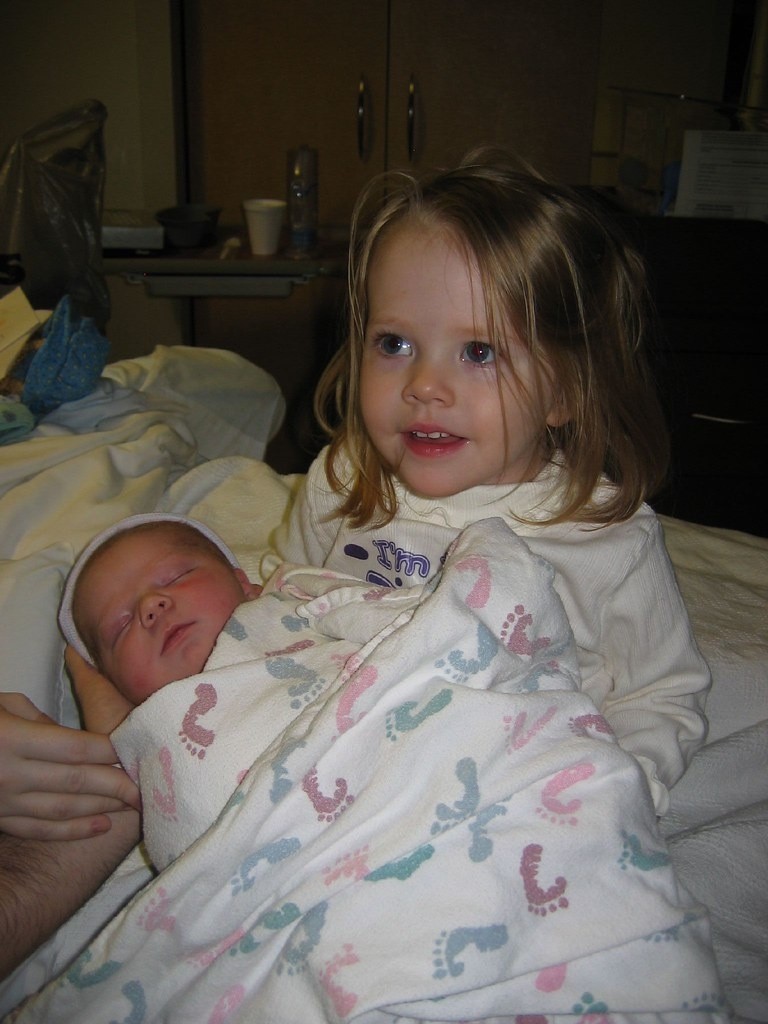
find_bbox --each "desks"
[103,224,362,348]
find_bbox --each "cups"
[285,149,320,255]
[243,198,287,256]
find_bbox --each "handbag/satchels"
[0,98,112,339]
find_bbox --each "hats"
[57,511,243,669]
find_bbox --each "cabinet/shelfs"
[185,0,603,472]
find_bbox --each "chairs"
[0,98,111,337]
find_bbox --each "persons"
[0,641,142,978]
[1,516,736,1024]
[248,139,710,825]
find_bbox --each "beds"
[0,289,768,1024]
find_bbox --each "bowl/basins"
[154,204,222,247]
